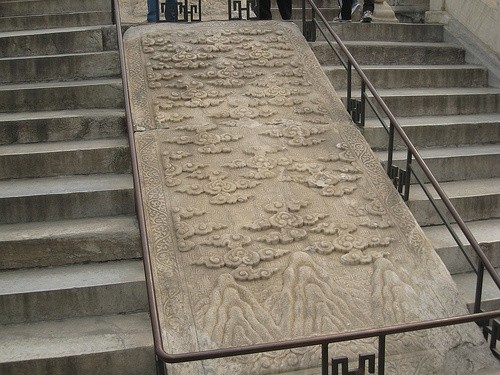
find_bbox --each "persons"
[139,0,179,24]
[333,0,376,22]
[258,1,295,22]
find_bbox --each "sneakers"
[332,13,351,23]
[359,11,373,22]
